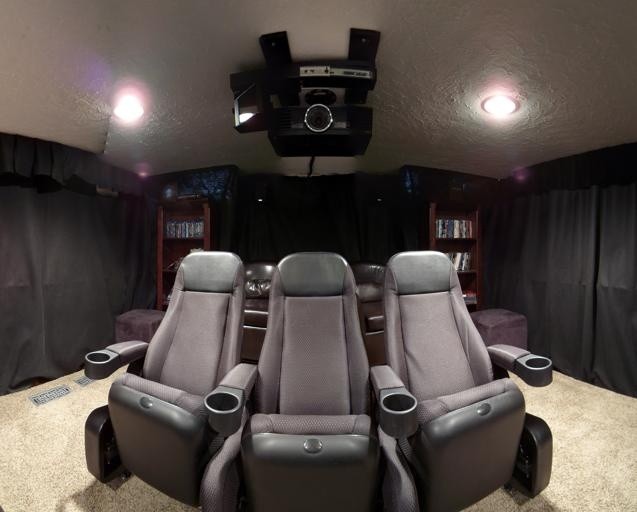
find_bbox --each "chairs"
[378,248,554,512]
[222,252,416,512]
[86,250,247,512]
[352,263,391,366]
[239,261,277,366]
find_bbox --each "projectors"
[268,103,373,157]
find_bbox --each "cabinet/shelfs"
[413,199,483,314]
[155,196,221,311]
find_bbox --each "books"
[435,217,477,306]
[164,217,204,271]
